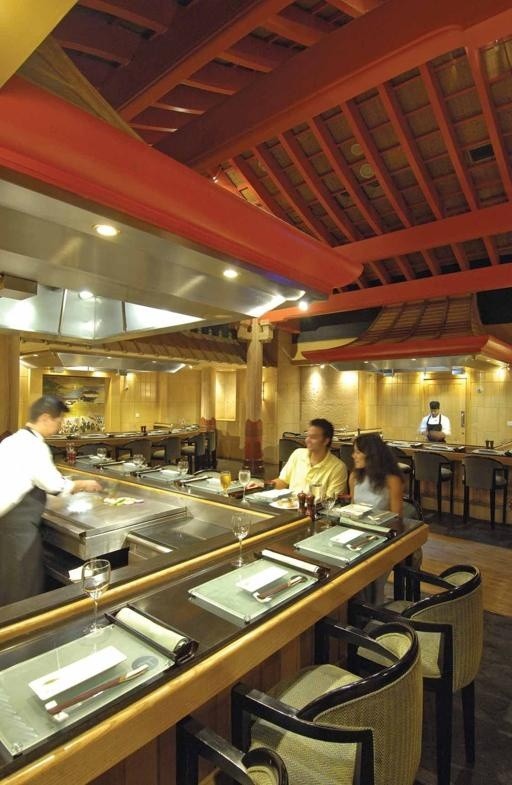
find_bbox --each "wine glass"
[239,471,250,504]
[133,453,146,482]
[320,490,335,529]
[177,460,189,490]
[220,469,232,498]
[230,512,255,568]
[80,559,111,638]
[97,448,108,470]
[309,478,322,519]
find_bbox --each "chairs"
[339,444,355,494]
[279,439,304,473]
[387,446,508,530]
[388,498,423,601]
[231,614,423,785]
[48,426,221,475]
[347,564,484,785]
[175,715,288,784]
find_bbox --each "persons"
[243,417,349,503]
[344,431,405,633]
[417,400,451,443]
[0,395,105,607]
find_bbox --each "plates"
[270,497,307,512]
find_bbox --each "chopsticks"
[257,576,302,599]
[47,664,148,716]
[351,536,376,549]
